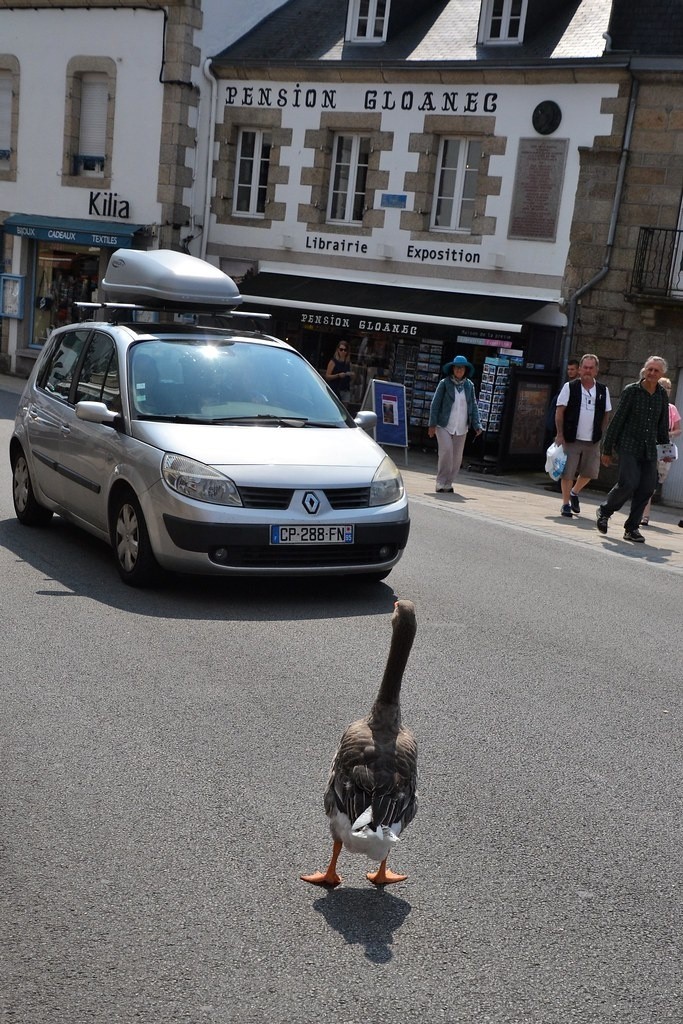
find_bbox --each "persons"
[555,354,612,517]
[428,355,482,493]
[595,355,673,543]
[325,340,354,413]
[637,377,682,525]
[543,359,579,493]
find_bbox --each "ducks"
[298,601,418,887]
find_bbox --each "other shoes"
[640,517,648,525]
[435,483,454,493]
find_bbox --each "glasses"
[338,347,348,352]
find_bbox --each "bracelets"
[338,374,340,378]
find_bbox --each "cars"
[8,318,414,589]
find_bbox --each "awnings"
[4,213,146,249]
[230,272,566,336]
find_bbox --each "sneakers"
[561,504,572,517]
[623,530,645,542]
[595,507,610,535]
[569,491,580,513]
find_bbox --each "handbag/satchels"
[545,442,567,482]
[545,394,561,436]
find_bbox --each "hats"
[443,356,474,378]
